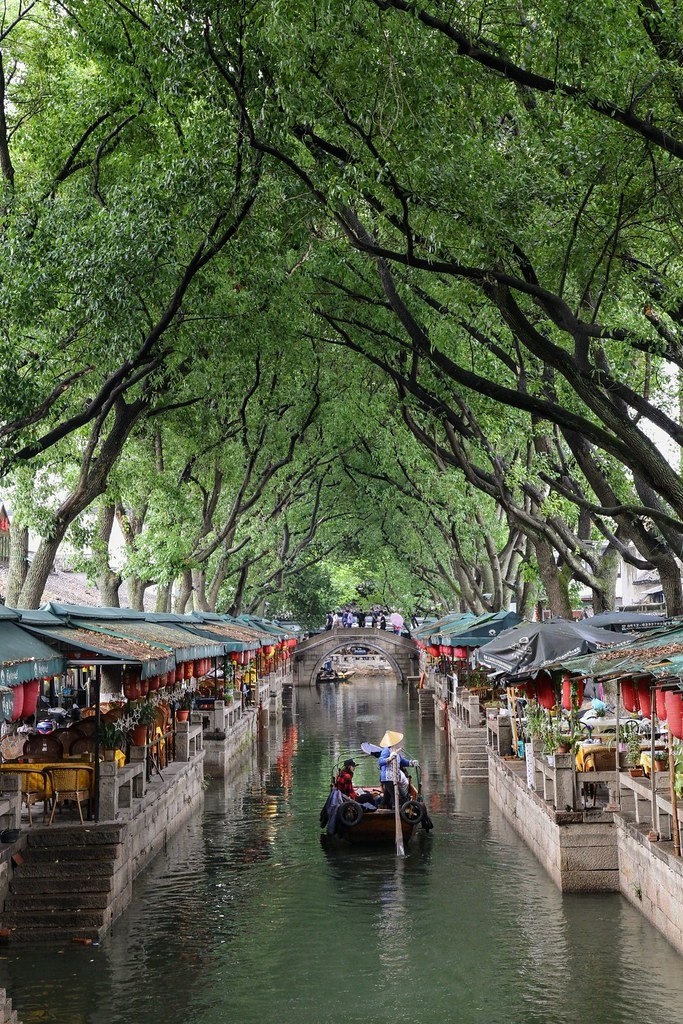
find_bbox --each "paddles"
[392,746,406,857]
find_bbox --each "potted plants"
[483,701,505,717]
[607,719,640,753]
[222,692,234,706]
[655,752,669,770]
[225,666,234,680]
[554,727,580,757]
[626,733,644,776]
[224,681,238,694]
[98,722,133,761]
[126,704,156,746]
[523,706,545,751]
[540,727,555,766]
[176,689,201,722]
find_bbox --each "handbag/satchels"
[355,790,375,807]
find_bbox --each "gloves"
[387,752,398,763]
[409,759,419,766]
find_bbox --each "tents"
[0,603,298,823]
[410,612,683,841]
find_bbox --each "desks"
[1,758,103,768]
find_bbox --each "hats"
[380,730,403,747]
[344,759,359,767]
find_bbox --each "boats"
[329,746,423,850]
[316,666,356,683]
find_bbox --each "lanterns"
[521,674,682,742]
[412,637,468,659]
[124,639,296,703]
[10,679,39,721]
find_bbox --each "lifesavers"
[338,800,362,827]
[401,800,424,824]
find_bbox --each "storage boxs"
[581,745,616,770]
[81,752,92,763]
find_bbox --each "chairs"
[0,679,223,827]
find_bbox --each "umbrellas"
[390,613,404,628]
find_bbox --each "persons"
[335,759,373,803]
[16,708,83,734]
[326,658,334,673]
[378,729,419,806]
[324,608,402,636]
